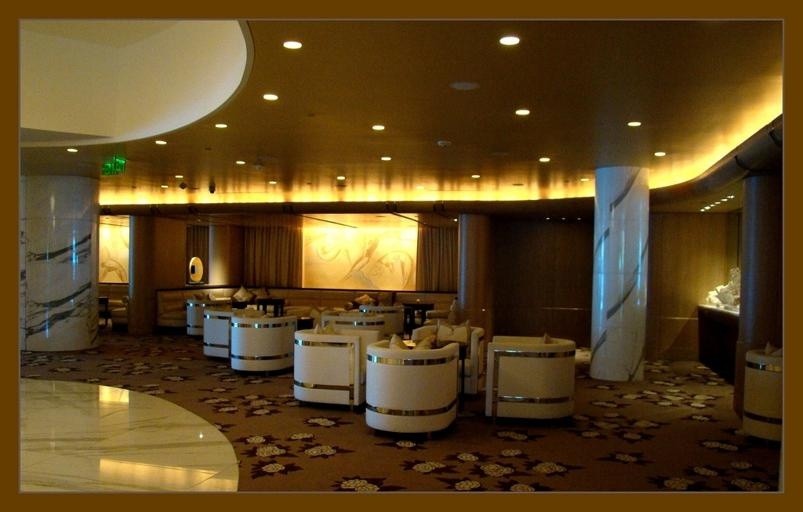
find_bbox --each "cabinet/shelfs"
[697,304,739,385]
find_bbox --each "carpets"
[18,337,780,493]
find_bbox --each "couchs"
[365,340,459,443]
[320,312,385,339]
[203,307,241,364]
[156,285,457,334]
[186,298,230,341]
[229,314,297,378]
[413,309,451,326]
[358,304,403,338]
[410,325,485,401]
[484,335,575,428]
[283,305,311,318]
[108,299,123,318]
[293,327,382,414]
[111,295,128,330]
[741,350,782,451]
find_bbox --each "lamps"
[20,19,254,149]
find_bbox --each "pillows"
[388,333,435,351]
[232,308,265,318]
[231,286,256,302]
[251,288,271,302]
[763,341,782,357]
[354,294,375,307]
[314,322,337,335]
[436,325,470,348]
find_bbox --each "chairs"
[97,297,109,326]
